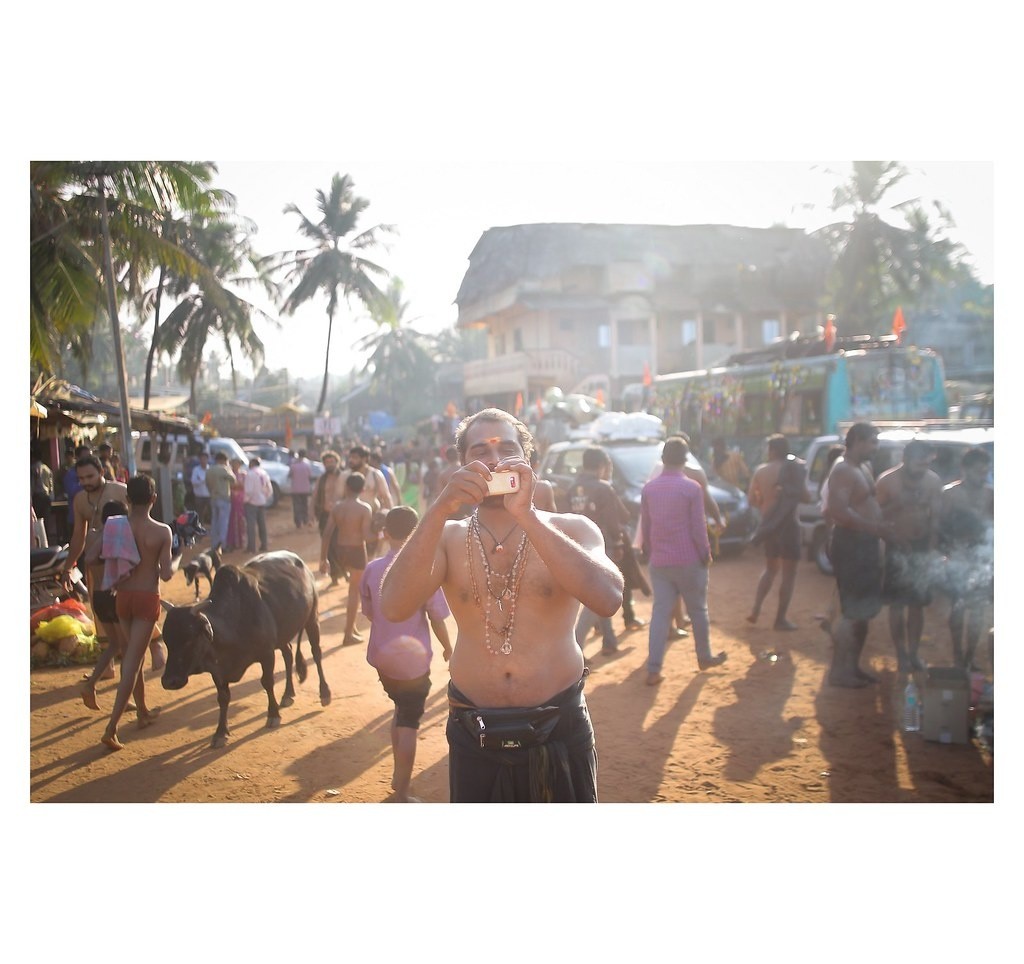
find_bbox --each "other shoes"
[677,617,693,630]
[626,618,648,631]
[666,627,689,640]
[602,643,616,656]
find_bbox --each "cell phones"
[483,469,520,496]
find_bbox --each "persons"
[377,407,624,806]
[61,436,464,804]
[532,419,994,691]
[30,438,54,518]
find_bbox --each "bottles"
[903,673,921,731]
[965,658,986,706]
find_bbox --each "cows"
[160,550,331,750]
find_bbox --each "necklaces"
[464,503,531,656]
[86,479,107,511]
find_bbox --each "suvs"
[793,423,992,579]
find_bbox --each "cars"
[133,432,328,507]
[538,441,758,563]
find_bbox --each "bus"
[643,346,949,506]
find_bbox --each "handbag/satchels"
[448,694,579,751]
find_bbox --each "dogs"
[168,511,208,561]
[182,542,223,603]
